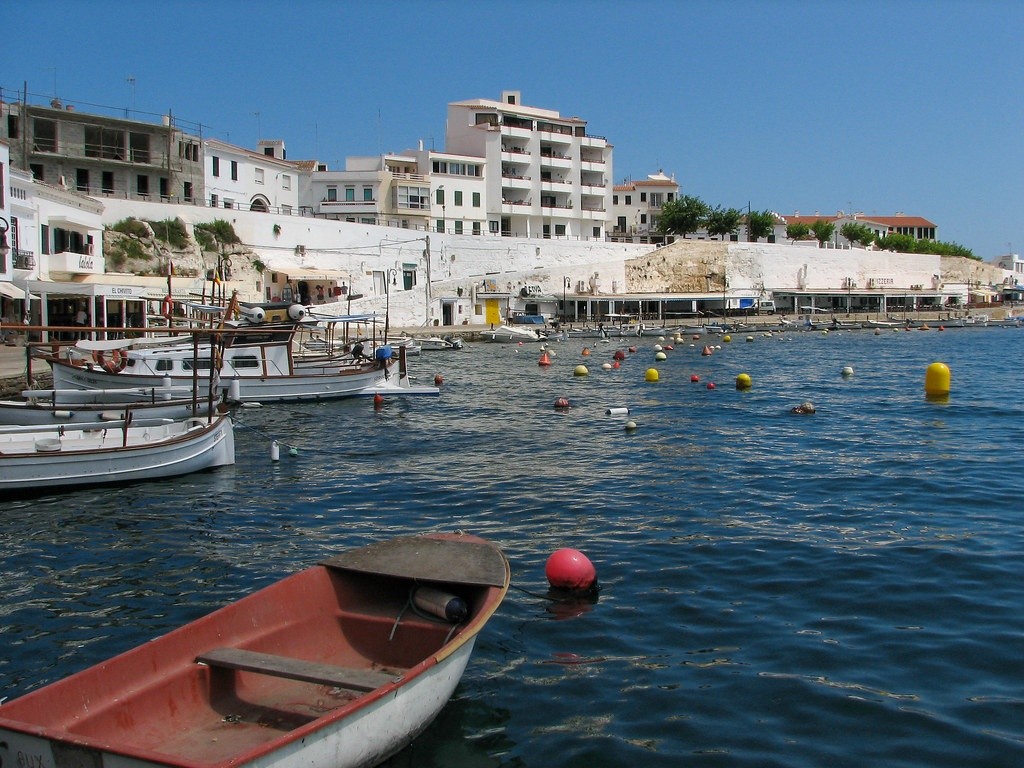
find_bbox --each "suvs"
[743,300,776,315]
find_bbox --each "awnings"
[562,292,760,301]
[267,267,352,280]
[773,288,964,298]
[476,293,519,298]
[0,281,42,300]
[521,295,561,303]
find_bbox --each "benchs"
[195,646,395,691]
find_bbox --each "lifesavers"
[162,295,173,320]
[97,346,128,374]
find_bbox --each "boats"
[0,402,236,502]
[0,525,506,768]
[479,325,550,344]
[20,208,465,409]
[540,313,1024,344]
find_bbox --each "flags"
[213,264,221,286]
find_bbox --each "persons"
[318,287,324,300]
[74,306,87,340]
[508,307,514,327]
[299,281,309,304]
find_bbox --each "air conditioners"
[870,278,877,288]
[846,277,852,286]
[577,280,587,292]
[84,243,94,256]
[297,245,306,254]
[17,255,33,268]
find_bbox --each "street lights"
[563,276,574,324]
[384,270,398,332]
[217,256,233,306]
[848,278,857,314]
[967,279,974,313]
[723,278,729,322]
